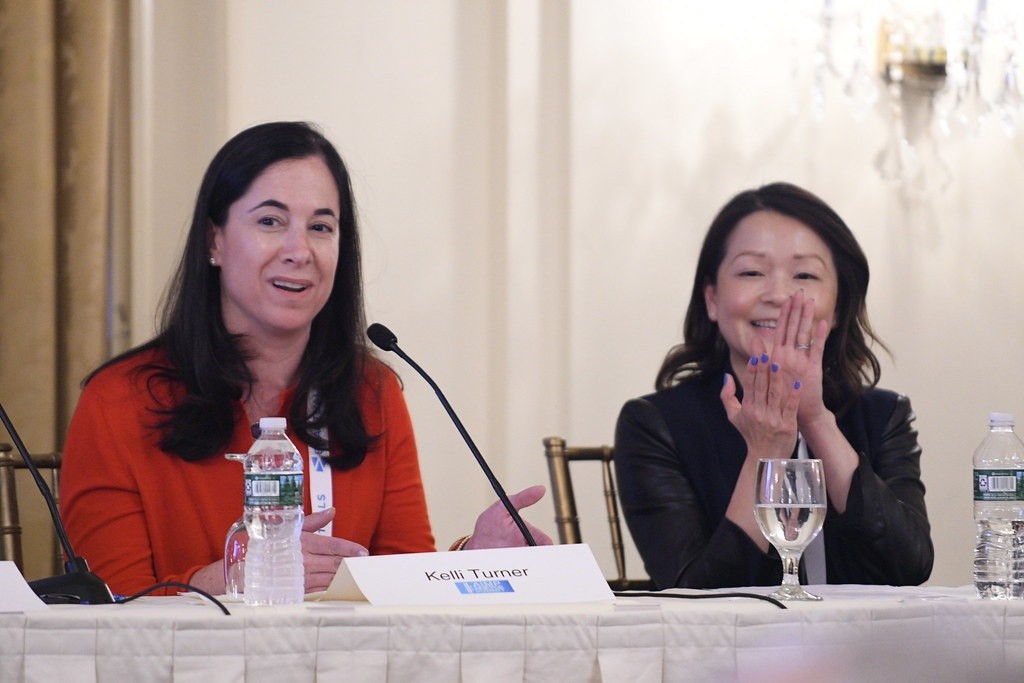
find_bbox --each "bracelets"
[457,535,473,551]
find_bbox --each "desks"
[0,581,1024,683]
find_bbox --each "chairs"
[0,443,62,581]
[544,437,651,591]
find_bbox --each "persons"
[59,122,554,598]
[614,182,935,589]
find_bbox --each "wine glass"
[754,459,827,602]
[223,453,244,601]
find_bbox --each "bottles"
[972,412,1024,600]
[243,417,304,605]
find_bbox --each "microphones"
[368,322,537,547]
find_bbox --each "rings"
[796,344,810,349]
